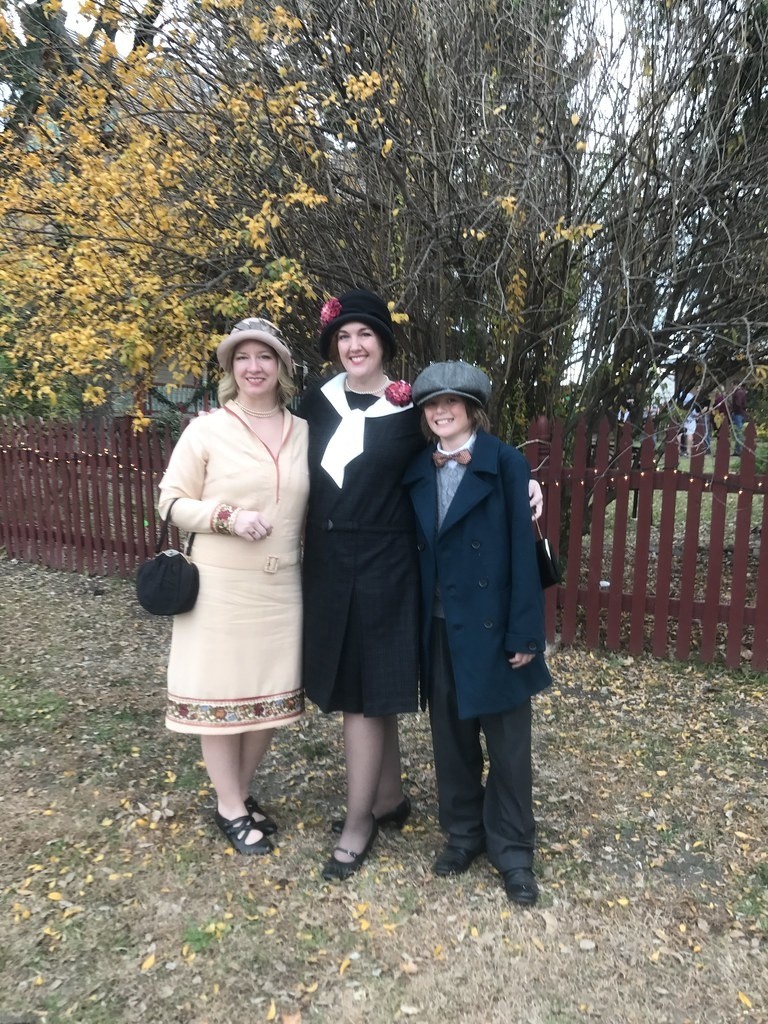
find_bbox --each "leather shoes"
[503,866,536,902]
[433,845,480,875]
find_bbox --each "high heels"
[323,811,377,880]
[331,796,411,833]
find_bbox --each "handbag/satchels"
[532,506,562,589]
[137,497,198,615]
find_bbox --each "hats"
[318,290,396,363]
[411,360,490,409]
[216,317,297,380]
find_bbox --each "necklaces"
[345,375,390,395]
[233,399,281,419]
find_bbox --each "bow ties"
[433,451,472,467]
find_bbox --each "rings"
[251,531,256,535]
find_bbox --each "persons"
[404,358,554,906]
[294,291,543,882]
[617,384,750,458]
[151,320,311,856]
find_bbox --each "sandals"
[243,796,277,837]
[216,808,273,855]
